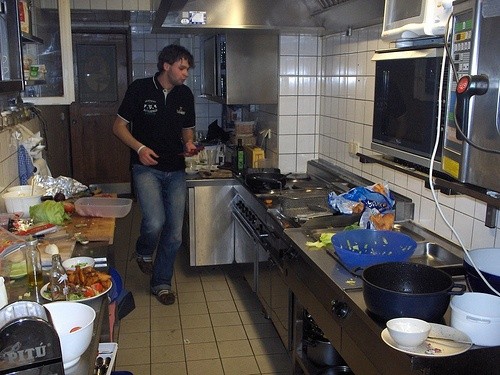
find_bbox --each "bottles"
[0,65,39,130]
[50,254,70,303]
[24,234,41,285]
[219,147,225,166]
[235,138,245,172]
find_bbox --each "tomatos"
[82,283,103,297]
[63,202,75,214]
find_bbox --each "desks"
[0,189,118,375]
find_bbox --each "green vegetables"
[44,292,83,300]
[305,225,361,248]
[71,263,87,267]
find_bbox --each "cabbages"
[29,200,72,225]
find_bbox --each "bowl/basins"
[1,190,45,220]
[63,256,95,272]
[7,185,44,193]
[41,302,96,370]
[332,228,500,349]
[74,197,132,218]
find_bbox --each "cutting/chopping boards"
[37,192,118,245]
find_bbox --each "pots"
[322,366,355,375]
[301,329,346,367]
[353,260,466,322]
[216,165,286,191]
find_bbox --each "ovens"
[225,192,271,295]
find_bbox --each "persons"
[113,44,196,304]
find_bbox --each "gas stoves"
[232,157,414,235]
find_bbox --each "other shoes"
[136,253,153,276]
[150,288,175,305]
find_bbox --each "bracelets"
[136,145,146,154]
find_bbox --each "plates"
[39,276,112,302]
[10,273,27,279]
[381,322,474,357]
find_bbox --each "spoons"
[93,356,112,375]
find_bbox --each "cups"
[0,277,8,310]
[186,149,218,172]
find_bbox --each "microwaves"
[381,0,454,40]
[371,46,451,178]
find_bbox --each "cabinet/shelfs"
[201,32,279,107]
